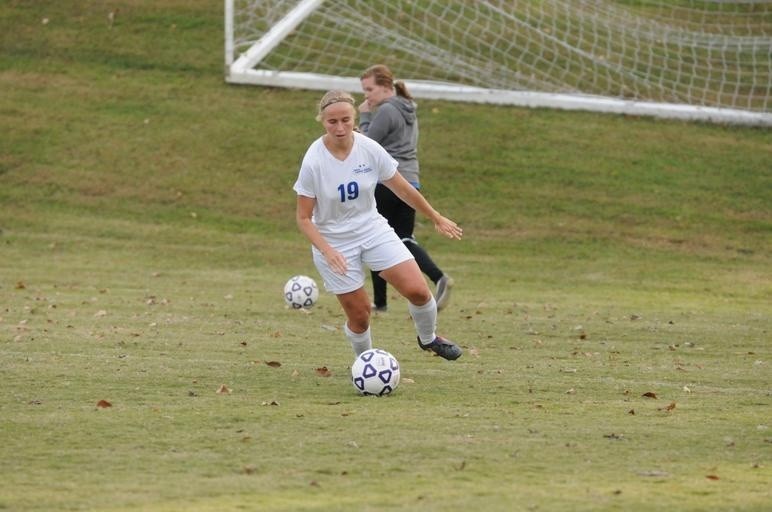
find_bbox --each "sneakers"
[416,335,462,362]
[434,272,454,312]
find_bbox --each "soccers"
[283,275,318,309]
[350,349,401,396]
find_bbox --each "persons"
[354,64,456,316]
[291,88,463,366]
